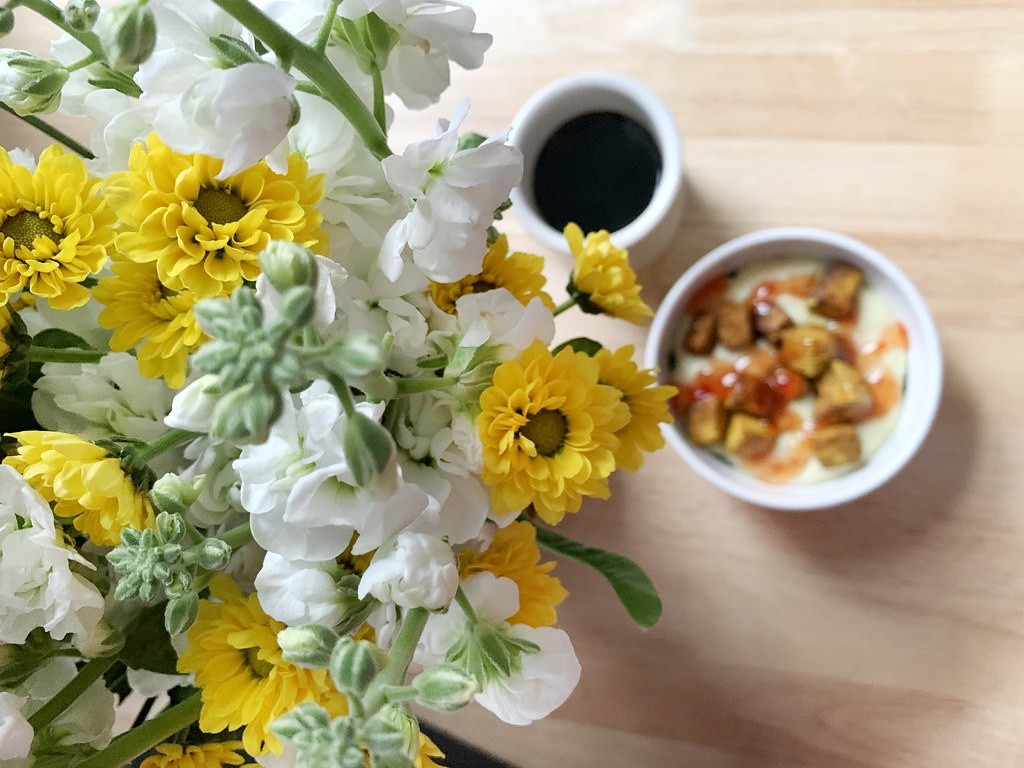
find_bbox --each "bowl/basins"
[638,225,947,515]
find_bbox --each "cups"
[506,75,684,273]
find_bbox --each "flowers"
[0,0,681,768]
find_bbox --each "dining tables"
[413,3,1023,767]
